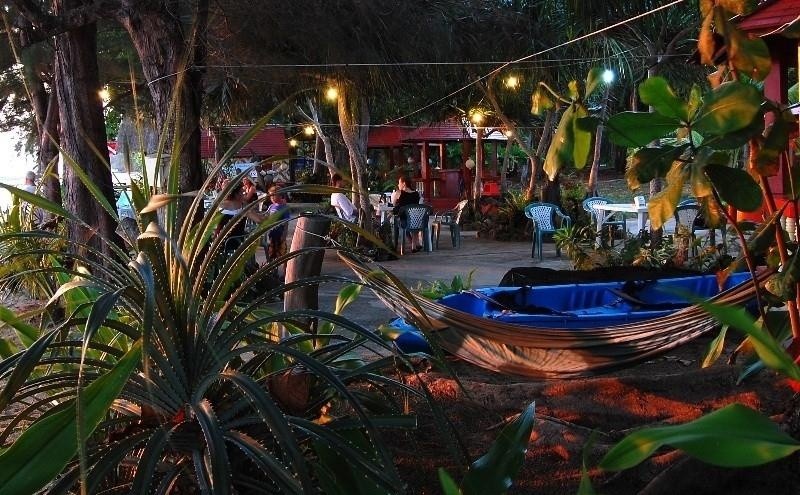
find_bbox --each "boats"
[379,272,768,355]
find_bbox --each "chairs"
[523,196,627,261]
[325,192,469,256]
[673,198,727,256]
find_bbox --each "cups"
[603,201,607,205]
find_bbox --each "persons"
[330,173,360,228]
[216,177,289,259]
[392,175,424,253]
[22,171,38,194]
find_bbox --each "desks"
[592,203,651,253]
